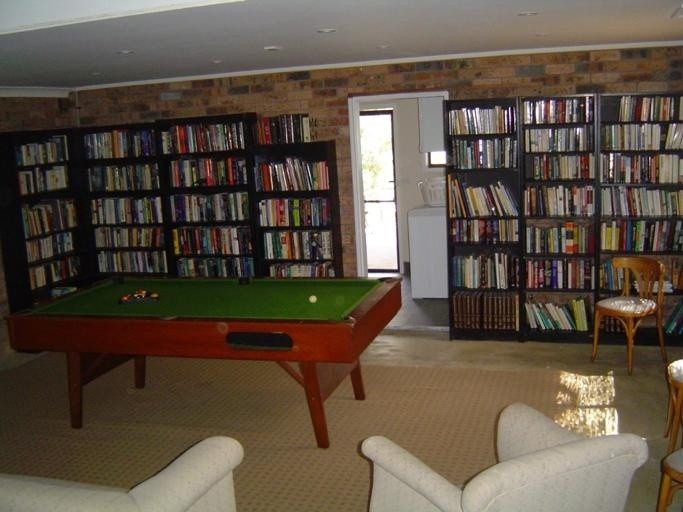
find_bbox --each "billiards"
[120,289,159,301]
[309,295,317,303]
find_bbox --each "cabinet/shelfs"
[444,92,678,343]
[9,114,344,311]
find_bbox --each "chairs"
[661,352,683,447]
[1,432,245,508]
[591,252,668,370]
[359,403,648,510]
[654,439,683,509]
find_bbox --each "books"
[600,96,682,333]
[449,106,520,333]
[83,130,167,274]
[523,96,594,333]
[256,114,337,279]
[161,120,255,280]
[19,134,80,290]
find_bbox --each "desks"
[5,269,403,447]
